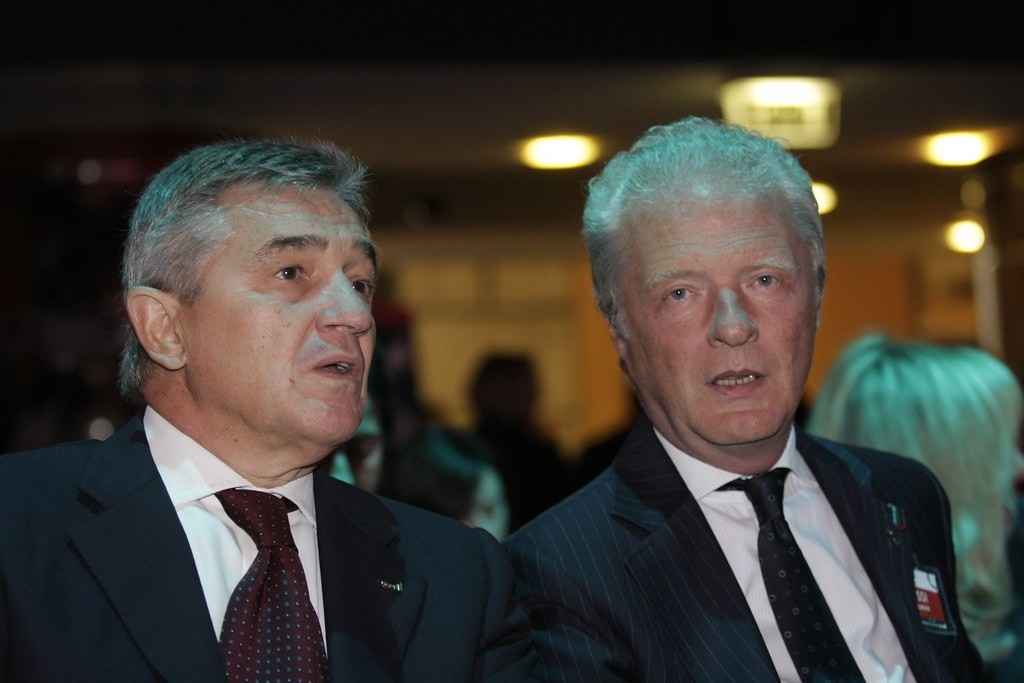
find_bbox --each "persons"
[0,134,512,683]
[471,348,572,536]
[499,113,996,683]
[804,332,1024,683]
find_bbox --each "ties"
[717,468,866,683]
[216,489,328,683]
